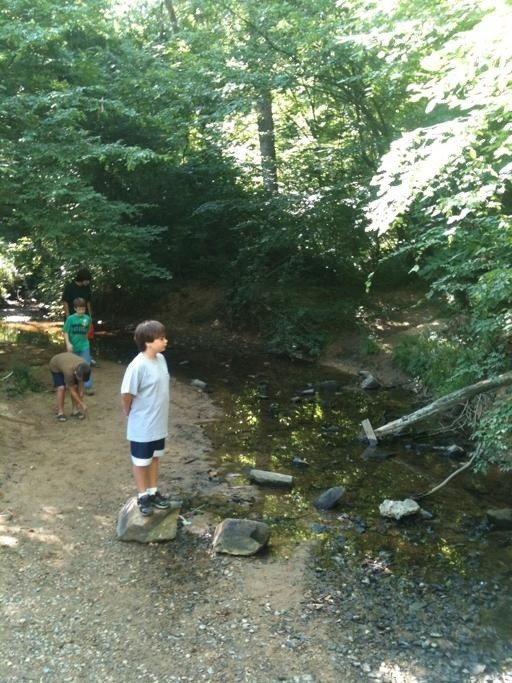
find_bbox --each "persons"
[62,267,98,367]
[47,352,92,422]
[120,320,171,517]
[63,296,96,396]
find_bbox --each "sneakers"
[148,491,172,509]
[84,388,96,396]
[71,411,85,419]
[136,494,154,517]
[57,413,67,422]
